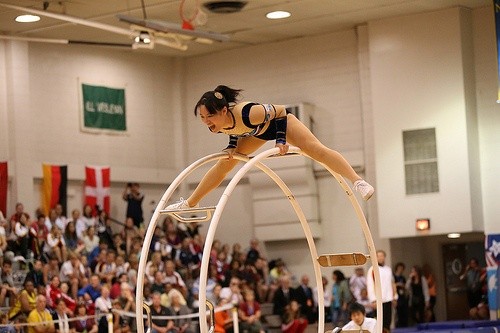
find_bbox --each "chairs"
[260,304,283,333]
[392,318,500,333]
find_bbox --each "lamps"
[417,219,430,230]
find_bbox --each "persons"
[162,84,376,210]
[0,180,439,333]
[460,257,487,319]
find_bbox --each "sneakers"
[352,180,374,201]
[165,197,199,210]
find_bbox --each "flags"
[82,165,112,220]
[42,164,70,218]
